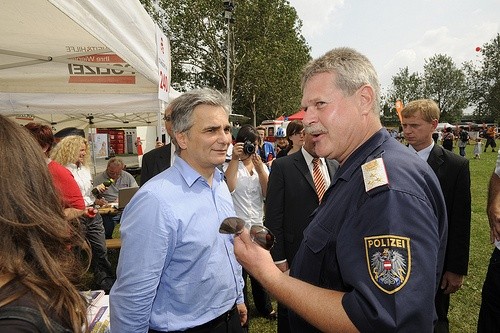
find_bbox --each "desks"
[98,202,123,215]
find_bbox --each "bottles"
[88,208,98,216]
[91,179,115,195]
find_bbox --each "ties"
[312,157,326,205]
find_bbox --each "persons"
[401,99,471,333]
[387,123,404,143]
[216,120,306,320]
[139,103,178,189]
[219,46,449,333]
[0,114,94,333]
[49,135,115,295]
[477,148,500,333]
[263,134,340,273]
[24,122,86,221]
[109,88,247,333]
[90,157,139,239]
[432,126,497,160]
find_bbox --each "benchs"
[82,239,122,255]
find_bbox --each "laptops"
[114,186,140,210]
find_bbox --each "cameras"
[241,141,256,155]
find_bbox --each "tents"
[435,123,456,129]
[0,0,171,128]
